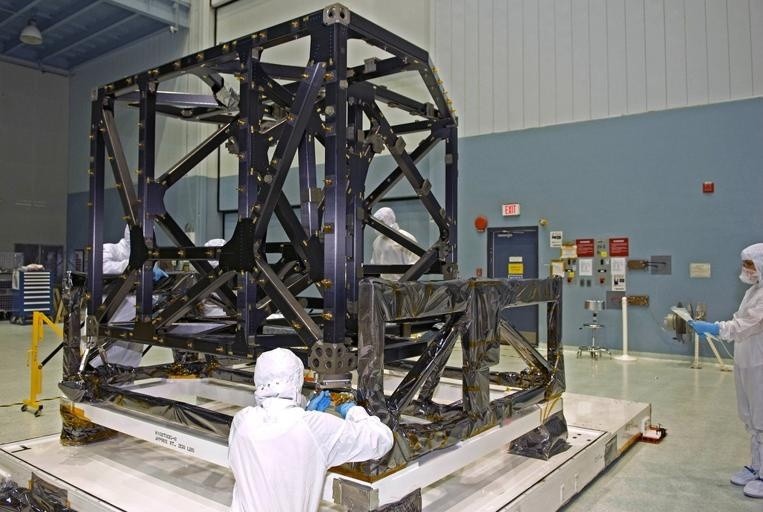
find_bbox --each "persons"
[369,207,420,282]
[229,347,395,511]
[688,241,763,498]
[199,238,238,315]
[75,222,169,383]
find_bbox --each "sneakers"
[731,467,762,497]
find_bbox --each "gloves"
[335,399,357,417]
[687,317,718,340]
[305,390,330,412]
[153,267,170,282]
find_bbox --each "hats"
[252,347,306,401]
[740,243,762,287]
[374,207,396,228]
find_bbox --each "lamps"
[585,300,605,327]
[20,18,43,45]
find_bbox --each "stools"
[576,323,612,360]
